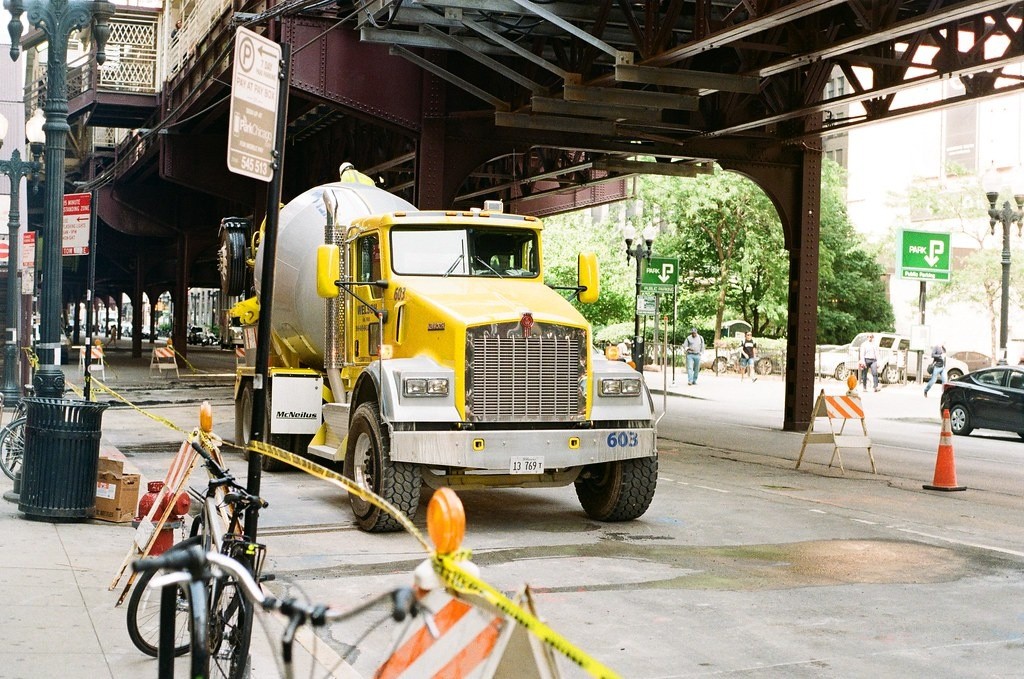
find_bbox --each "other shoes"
[874,389,880,392]
[688,382,692,385]
[863,389,867,392]
[693,381,696,384]
[753,377,757,382]
[924,392,927,398]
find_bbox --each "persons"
[860,332,879,392]
[482,250,510,275]
[683,329,704,384]
[739,331,757,383]
[171,20,187,69]
[105,325,118,348]
[924,338,948,398]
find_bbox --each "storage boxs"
[93,459,140,523]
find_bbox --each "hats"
[692,328,697,333]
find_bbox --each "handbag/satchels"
[927,364,934,374]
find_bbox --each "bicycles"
[127,441,278,679]
[0,382,38,483]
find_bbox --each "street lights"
[978,159,1022,371]
[0,107,50,407]
[624,217,658,364]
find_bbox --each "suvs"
[845,331,970,386]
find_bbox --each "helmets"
[339,162,354,176]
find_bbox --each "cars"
[940,364,1024,439]
[168,324,220,345]
[69,316,159,339]
[696,335,853,383]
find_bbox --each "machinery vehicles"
[217,179,663,533]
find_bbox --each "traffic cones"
[921,409,972,493]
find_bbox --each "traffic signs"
[900,229,952,273]
[643,257,679,287]
[62,192,94,258]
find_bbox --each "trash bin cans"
[18,396,110,522]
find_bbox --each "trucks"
[218,308,246,350]
